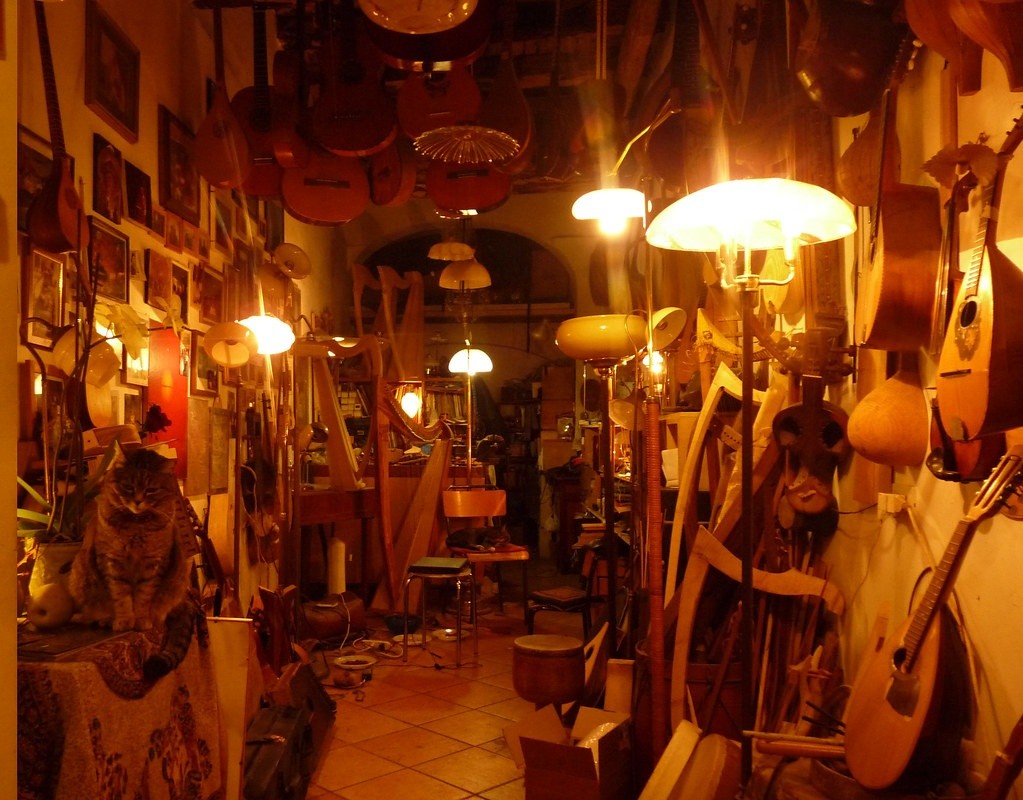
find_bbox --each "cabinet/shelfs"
[324,373,472,464]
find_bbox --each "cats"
[68,440,190,628]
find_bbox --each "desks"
[287,489,376,606]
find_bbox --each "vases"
[27,543,82,602]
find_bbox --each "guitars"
[25,0,89,254]
[843,443,1023,791]
[935,102,1023,441]
[852,24,941,352]
[192,1,725,228]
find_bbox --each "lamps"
[24,321,256,620]
[190,0,856,475]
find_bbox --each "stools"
[403,569,479,666]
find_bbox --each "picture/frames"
[86,135,302,496]
[79,0,141,145]
[158,104,202,224]
[21,244,67,353]
[16,121,75,238]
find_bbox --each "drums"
[511,633,586,721]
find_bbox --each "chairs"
[440,484,532,626]
[526,543,626,642]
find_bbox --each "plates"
[392,633,432,645]
[334,655,377,670]
[432,628,470,640]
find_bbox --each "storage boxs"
[502,701,629,800]
